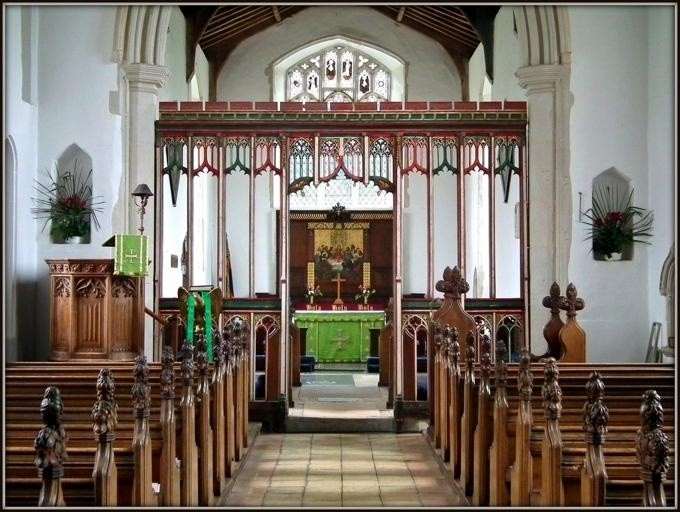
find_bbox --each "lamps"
[132,184,154,236]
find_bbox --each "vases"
[64,236,85,244]
[604,252,622,261]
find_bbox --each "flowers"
[576,184,655,258]
[30,157,107,240]
